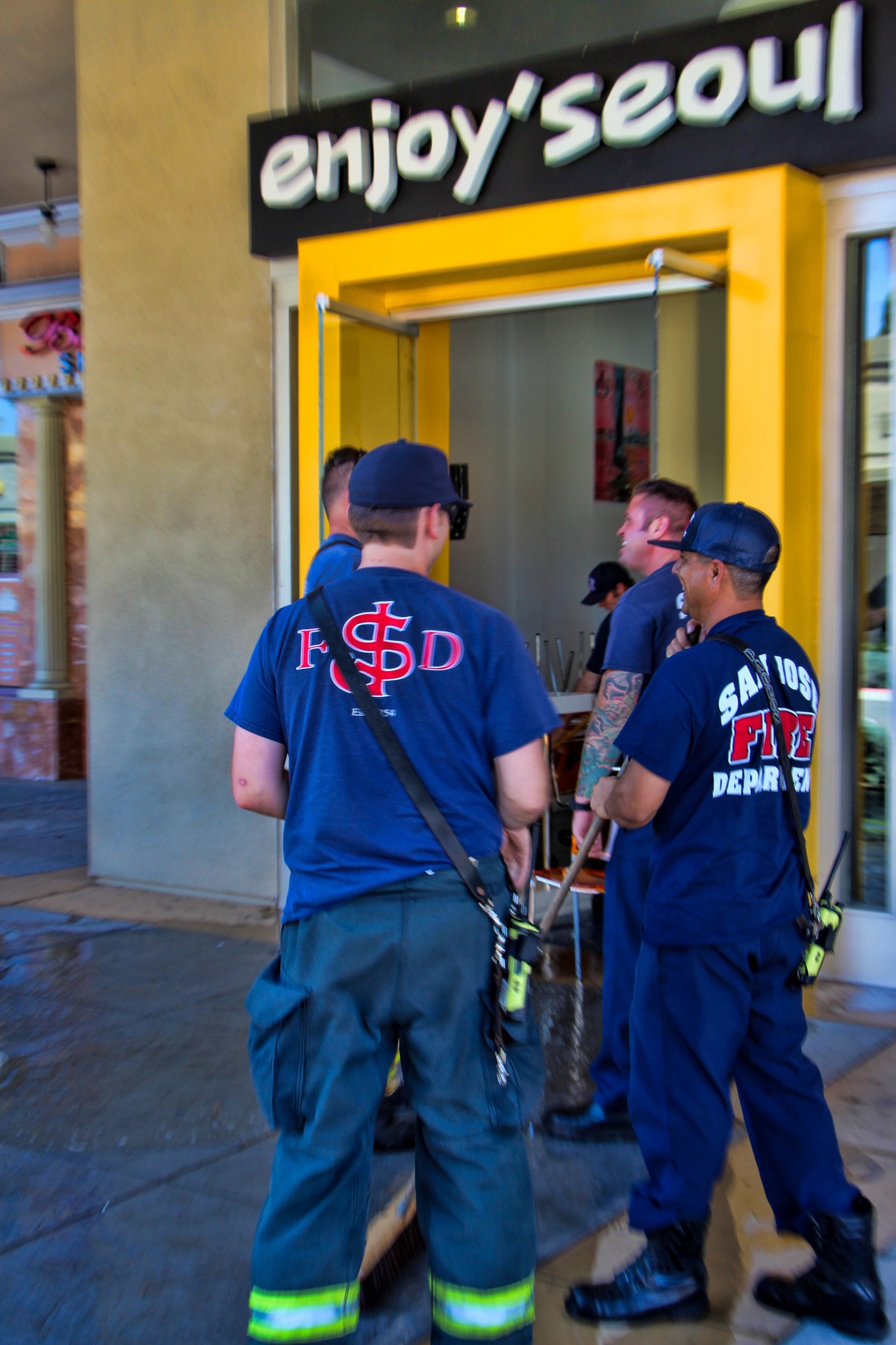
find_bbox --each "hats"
[647,502,781,573]
[580,561,629,605]
[348,439,475,509]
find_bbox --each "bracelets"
[568,793,592,811]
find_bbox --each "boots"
[564,1206,710,1325]
[753,1193,890,1339]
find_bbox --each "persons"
[224,443,562,1345]
[566,500,893,1343]
[304,446,698,1155]
[859,575,886,643]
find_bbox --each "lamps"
[35,156,60,249]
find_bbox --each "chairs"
[524,816,621,984]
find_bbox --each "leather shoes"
[374,1087,418,1152]
[544,1090,631,1138]
